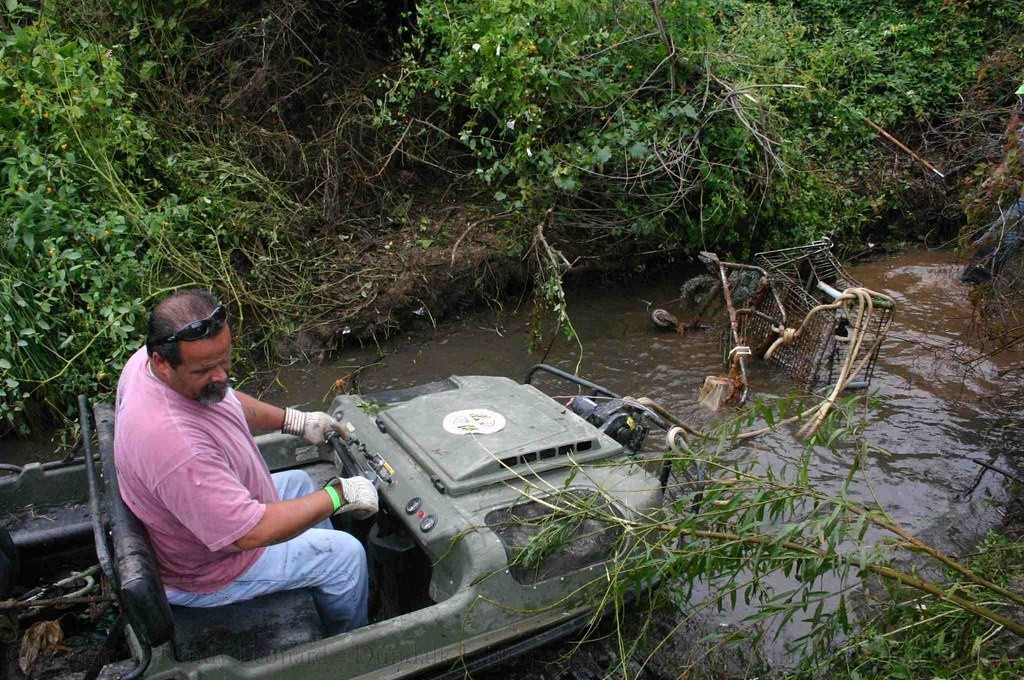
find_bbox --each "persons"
[115,288,380,635]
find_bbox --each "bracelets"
[323,485,341,513]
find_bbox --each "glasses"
[149,306,226,347]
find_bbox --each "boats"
[1,361,707,680]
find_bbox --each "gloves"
[324,476,379,519]
[282,407,350,446]
